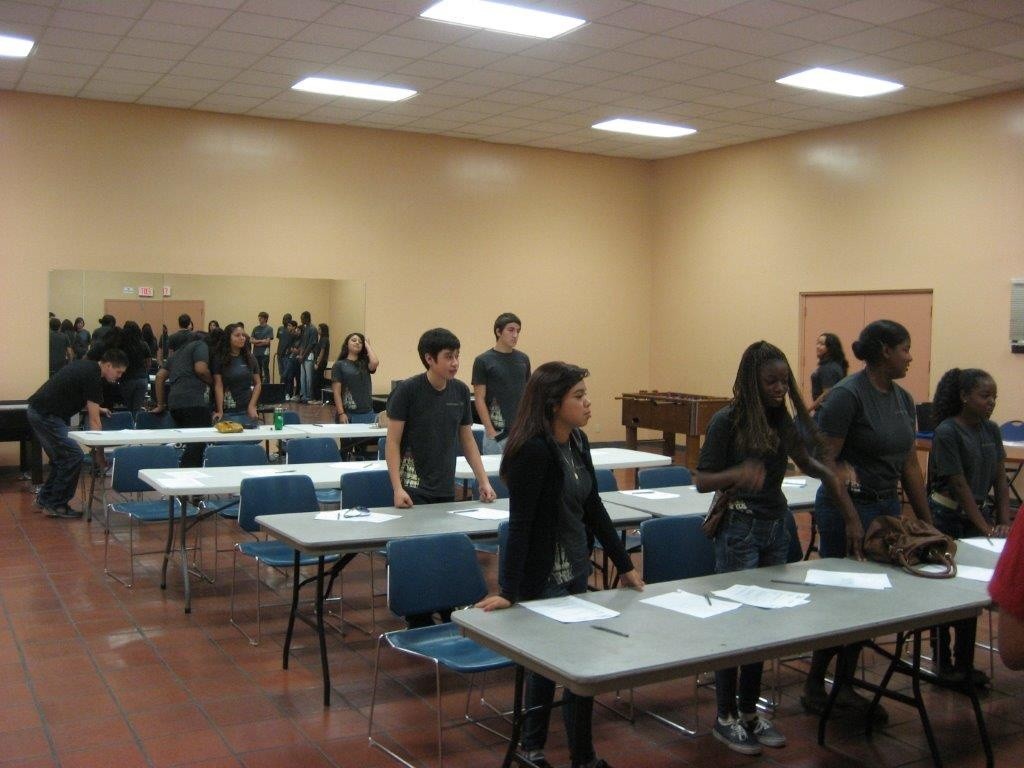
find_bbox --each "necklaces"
[558,438,579,480]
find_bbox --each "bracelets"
[986,526,996,537]
[157,401,167,407]
[338,411,346,416]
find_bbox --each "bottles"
[274,408,283,430]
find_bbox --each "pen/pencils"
[781,481,806,485]
[337,512,341,520]
[987,537,994,546]
[84,432,103,435]
[270,427,273,431]
[275,470,296,474]
[362,463,373,469]
[703,591,712,607]
[453,509,480,514]
[312,424,323,427]
[173,429,182,433]
[770,579,817,588]
[631,491,655,494]
[589,624,629,639]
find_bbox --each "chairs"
[101,444,204,588]
[590,467,641,587]
[273,412,301,455]
[367,531,524,768]
[286,438,343,510]
[135,411,175,430]
[80,411,140,514]
[256,383,287,425]
[346,414,379,460]
[638,464,693,489]
[614,515,777,737]
[193,445,271,584]
[480,521,635,726]
[230,475,346,648]
[998,418,1024,504]
[328,470,395,636]
[214,414,258,429]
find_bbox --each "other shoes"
[182,495,204,502]
[801,693,844,719]
[571,759,611,768]
[832,693,890,719]
[959,670,990,685]
[932,663,964,680]
[517,754,551,768]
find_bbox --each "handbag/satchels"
[863,516,957,579]
[216,420,243,433]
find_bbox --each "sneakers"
[743,716,786,746]
[43,504,83,518]
[712,715,761,754]
[31,497,45,511]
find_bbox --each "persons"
[383,325,497,632]
[471,312,531,456]
[49,307,329,417]
[987,502,1024,670]
[212,324,262,422]
[475,360,648,767]
[815,320,934,722]
[925,367,1014,689]
[331,332,379,461]
[148,330,216,509]
[695,340,842,755]
[805,332,850,422]
[26,347,129,519]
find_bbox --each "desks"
[285,421,486,460]
[0,400,46,493]
[135,460,389,615]
[598,474,823,561]
[69,422,308,535]
[454,447,672,502]
[844,536,1023,768]
[255,497,651,707]
[450,558,994,768]
